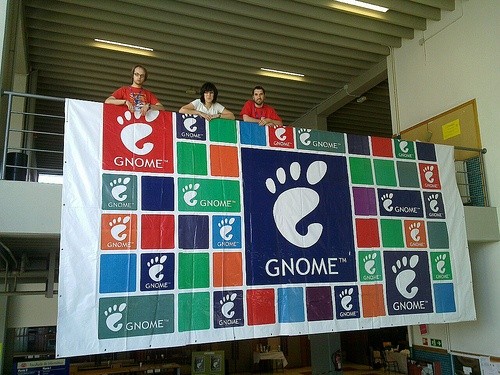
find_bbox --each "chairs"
[110,363,124,375]
[385,351,401,375]
[368,346,386,370]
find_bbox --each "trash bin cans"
[6,152,28,180]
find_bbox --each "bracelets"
[149,103,151,109]
[218,113,221,118]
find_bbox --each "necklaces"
[129,85,143,105]
[253,102,263,120]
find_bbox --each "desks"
[254,350,289,373]
[385,349,410,374]
[78,363,184,375]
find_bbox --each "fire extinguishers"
[420,325,427,334]
[332,350,342,371]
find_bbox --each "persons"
[179,82,235,121]
[239,86,283,128]
[105,64,166,115]
